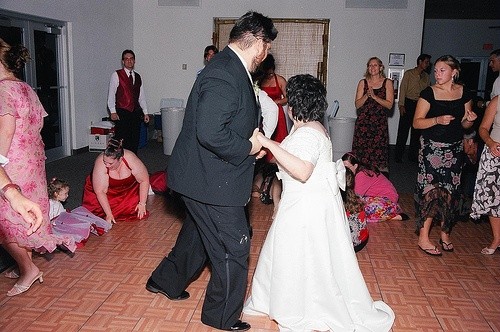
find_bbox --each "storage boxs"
[89,134,111,149]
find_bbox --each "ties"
[129,71,133,85]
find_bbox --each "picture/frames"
[389,53,406,66]
[388,68,405,103]
[343,0,409,10]
[156,0,202,8]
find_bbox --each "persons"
[203,46,219,63]
[471,94,500,255]
[412,56,477,255]
[254,73,378,332]
[256,54,289,166]
[339,167,370,253]
[146,8,268,332]
[341,151,410,223]
[82,140,150,226]
[395,54,433,164]
[108,49,149,156]
[0,155,43,235]
[0,37,52,297]
[488,49,500,99]
[47,176,104,252]
[352,57,395,178]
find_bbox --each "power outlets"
[182,64,187,70]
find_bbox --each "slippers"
[440,238,453,252]
[418,244,442,256]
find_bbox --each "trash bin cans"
[329,116,357,163]
[161,106,186,156]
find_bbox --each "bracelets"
[139,201,146,206]
[0,182,21,201]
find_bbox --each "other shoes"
[399,213,410,221]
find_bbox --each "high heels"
[5,269,20,278]
[6,271,44,296]
[481,244,500,254]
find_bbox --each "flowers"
[253,80,262,96]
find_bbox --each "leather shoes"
[201,320,251,332]
[146,276,190,300]
